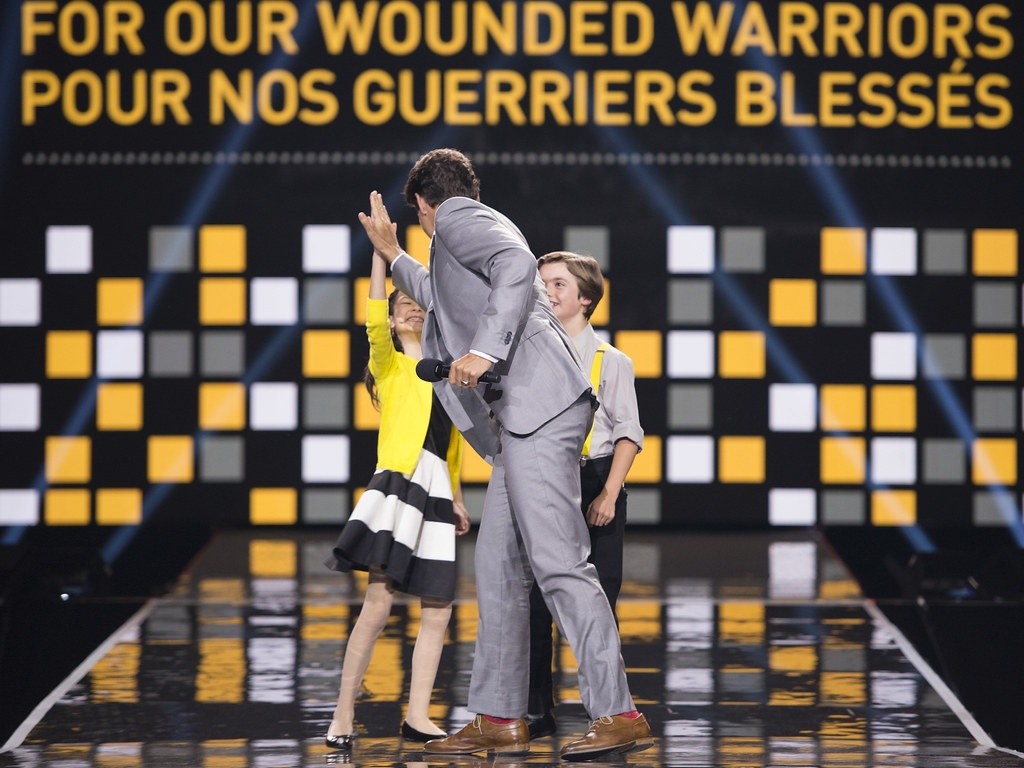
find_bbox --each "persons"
[325,148,655,761]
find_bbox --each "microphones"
[415,358,501,383]
[398,317,405,323]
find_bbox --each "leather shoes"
[524,712,557,740]
[424,714,530,753]
[561,713,655,760]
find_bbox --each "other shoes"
[326,734,352,750]
[402,720,447,742]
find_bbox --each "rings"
[461,380,470,387]
